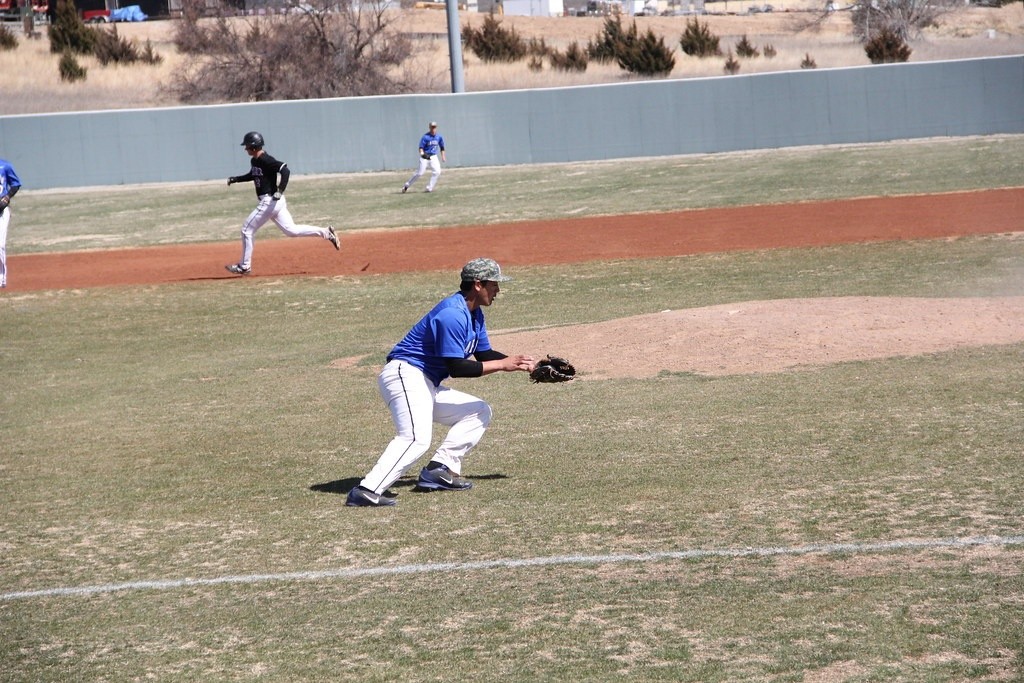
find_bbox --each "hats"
[429,121,437,127]
[461,257,513,282]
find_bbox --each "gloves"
[227,176,235,186]
[0,195,10,208]
[272,192,281,200]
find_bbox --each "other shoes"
[402,183,408,193]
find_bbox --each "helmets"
[240,132,265,146]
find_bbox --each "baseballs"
[404,182,410,188]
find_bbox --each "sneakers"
[346,487,397,506]
[328,224,341,250]
[225,264,251,275]
[418,465,472,490]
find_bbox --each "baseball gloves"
[421,153,430,160]
[529,356,577,384]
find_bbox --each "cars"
[749,4,773,13]
[633,6,658,16]
[400,0,467,12]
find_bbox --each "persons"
[402,122,446,194]
[0,158,22,288]
[225,132,340,276]
[345,257,577,507]
